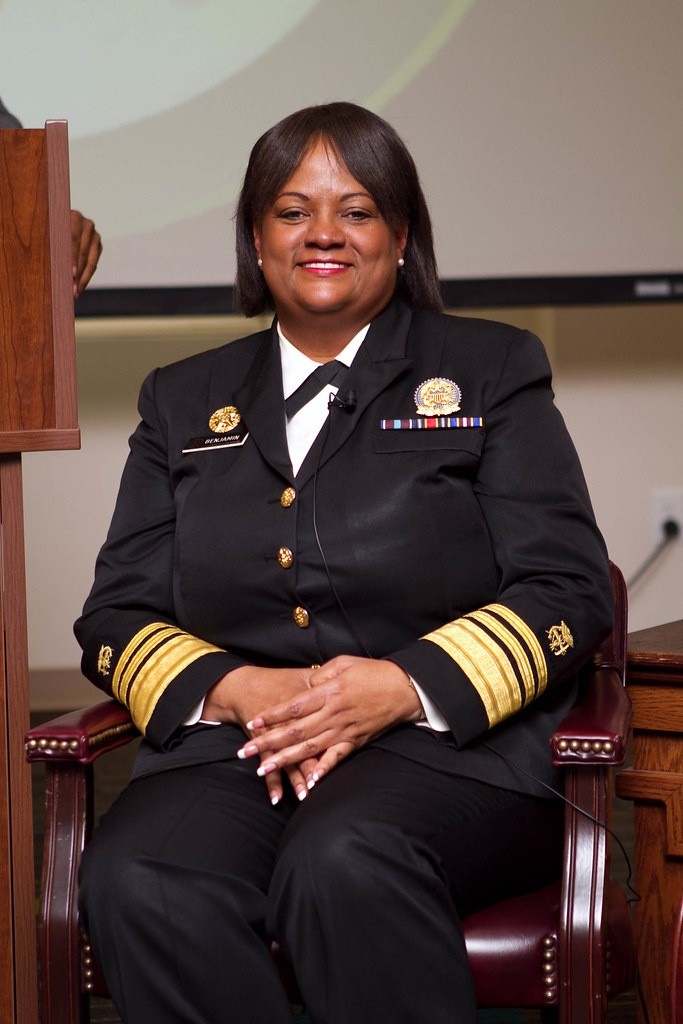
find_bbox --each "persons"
[74,101,614,1024]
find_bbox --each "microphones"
[627,518,679,594]
[328,387,358,414]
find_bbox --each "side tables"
[605,616,683,1024]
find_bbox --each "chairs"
[19,558,630,1023]
